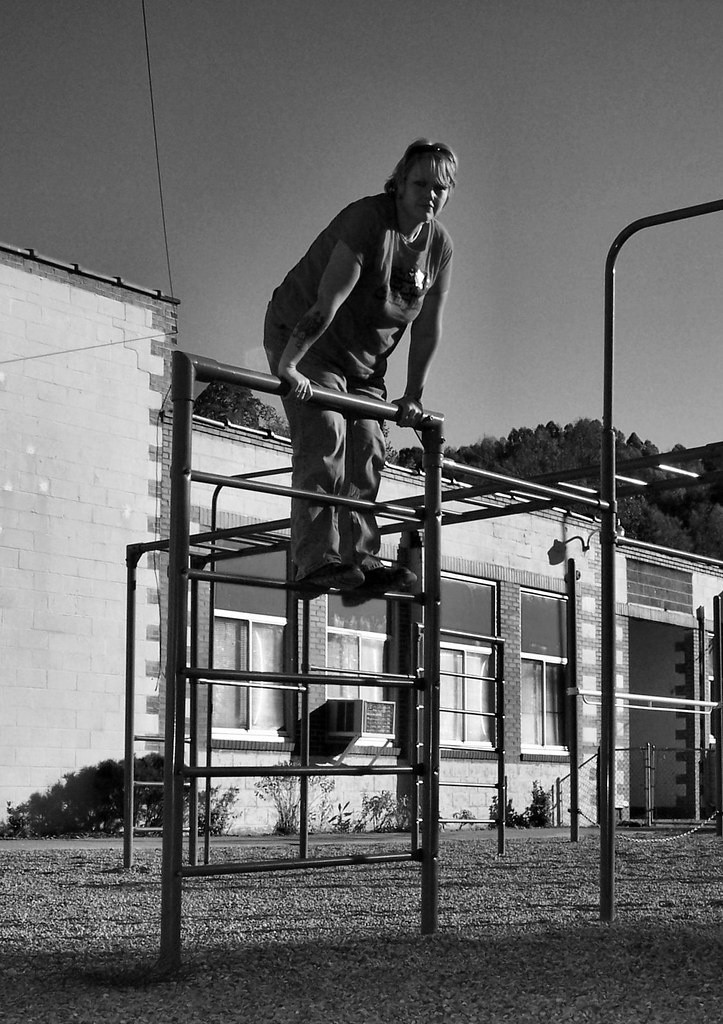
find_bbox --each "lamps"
[585,518,625,550]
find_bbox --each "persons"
[263,137,458,608]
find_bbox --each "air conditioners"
[326,699,395,740]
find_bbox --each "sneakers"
[342,565,417,607]
[291,562,365,600]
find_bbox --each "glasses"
[405,144,456,166]
